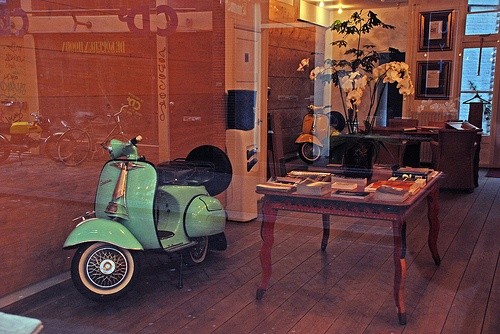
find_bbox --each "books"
[256,166,438,202]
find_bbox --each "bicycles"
[43,104,131,168]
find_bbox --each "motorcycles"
[0,112,55,165]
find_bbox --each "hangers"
[463,90,491,105]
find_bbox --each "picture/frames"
[414,58,453,101]
[416,7,455,52]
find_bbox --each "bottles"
[227,89,256,131]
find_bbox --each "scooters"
[295,104,379,175]
[60,135,233,302]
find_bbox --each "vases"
[342,132,375,180]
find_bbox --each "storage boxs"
[9,121,30,135]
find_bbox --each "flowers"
[296,8,414,137]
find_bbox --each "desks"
[250,160,448,328]
[374,122,484,193]
